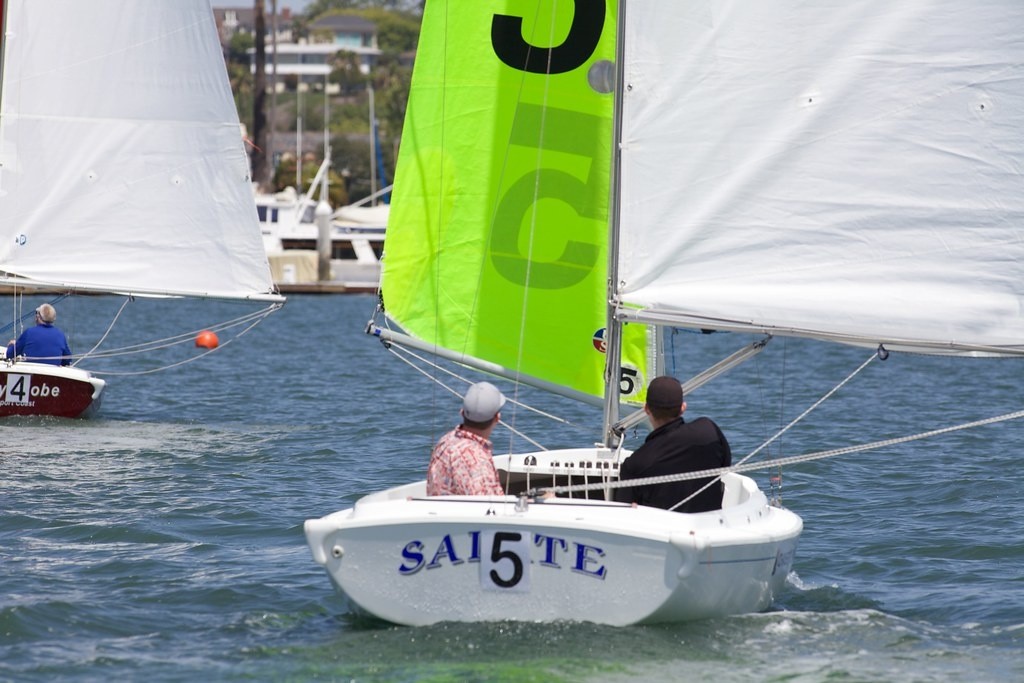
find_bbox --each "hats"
[464,382,507,422]
[646,376,684,408]
[36,304,56,322]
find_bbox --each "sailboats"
[302,3,1023,629]
[0,2,287,419]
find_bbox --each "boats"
[253,63,391,291]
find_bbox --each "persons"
[427,382,555,497]
[617,376,732,513]
[6,303,72,365]
